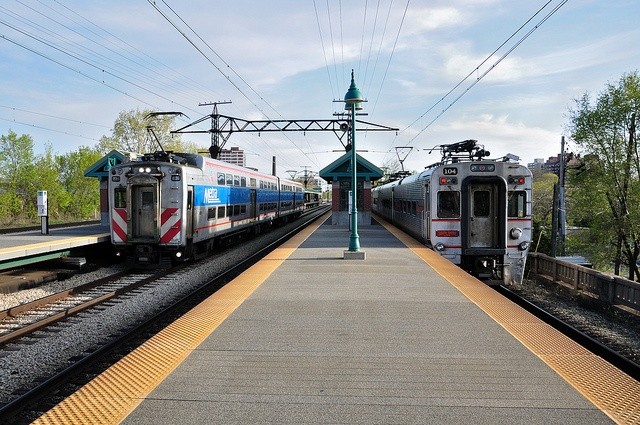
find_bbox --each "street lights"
[343,68,365,259]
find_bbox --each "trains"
[371,139,537,289]
[106,112,325,267]
